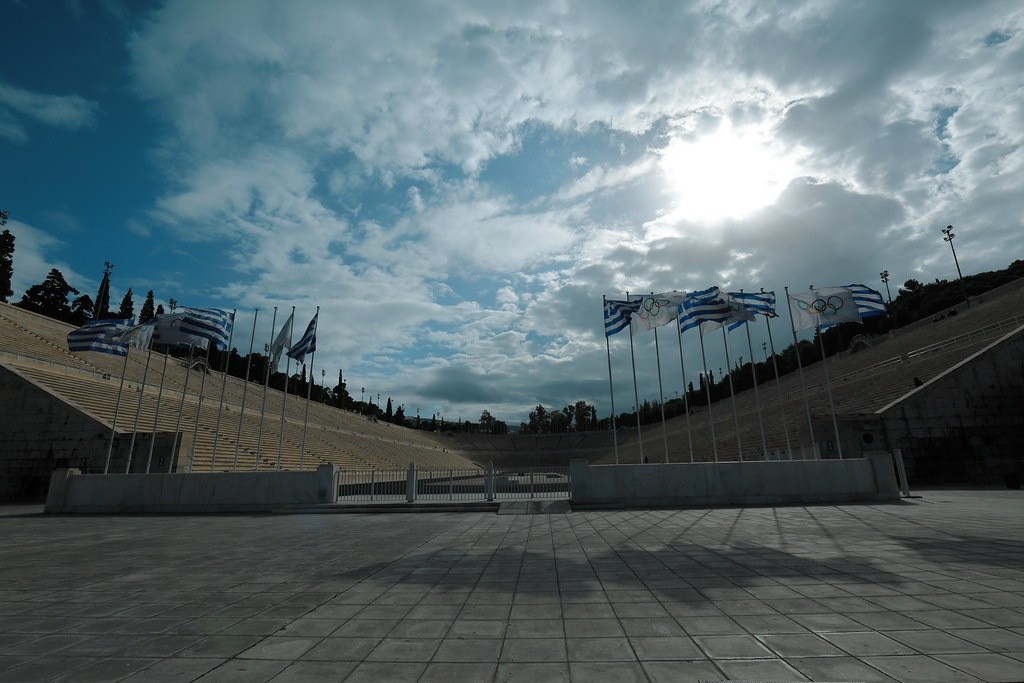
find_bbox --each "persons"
[912,377,923,387]
[932,307,958,322]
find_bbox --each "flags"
[815,283,887,329]
[178,306,236,349]
[155,312,208,350]
[108,319,157,352]
[270,313,293,375]
[285,312,318,362]
[786,287,864,333]
[603,298,642,337]
[627,291,686,335]
[67,319,135,355]
[674,286,781,336]
